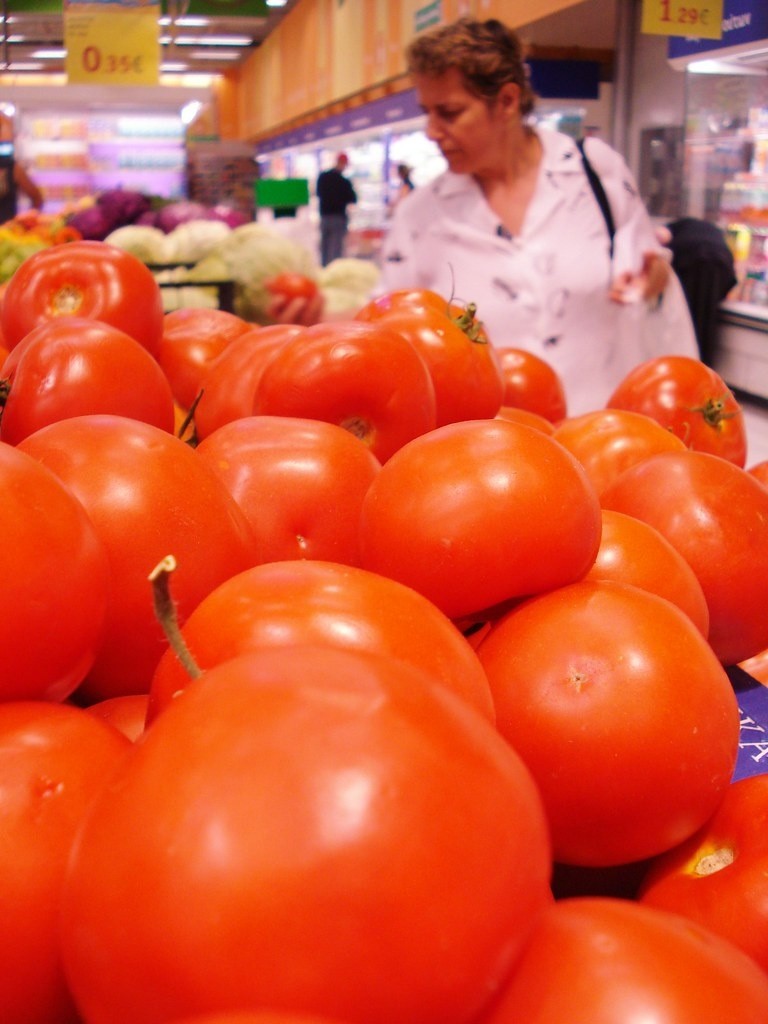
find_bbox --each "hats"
[339,154,351,167]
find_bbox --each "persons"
[384,16,698,419]
[316,151,415,270]
[0,140,43,224]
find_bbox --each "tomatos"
[0,242,768,1024]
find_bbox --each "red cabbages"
[64,189,248,241]
